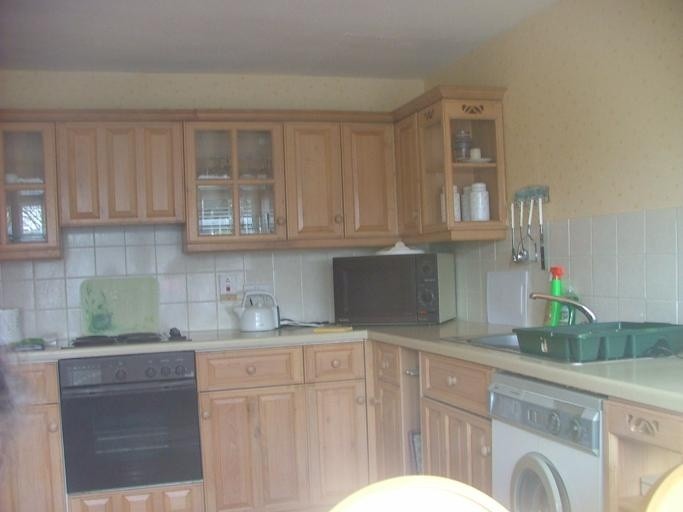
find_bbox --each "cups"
[469,148,482,160]
[195,165,274,236]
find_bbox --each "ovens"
[59,378,218,496]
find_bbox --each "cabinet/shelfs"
[420,349,493,495]
[55,119,186,228]
[179,119,288,250]
[192,344,311,510]
[282,123,399,248]
[392,112,421,246]
[365,341,423,483]
[413,95,508,242]
[298,333,376,510]
[0,362,68,512]
[0,123,62,260]
[600,399,681,511]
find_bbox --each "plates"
[468,158,492,163]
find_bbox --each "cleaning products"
[543,265,564,326]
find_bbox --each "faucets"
[530,290,597,323]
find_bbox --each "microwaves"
[331,253,459,327]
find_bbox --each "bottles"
[454,120,471,163]
[454,179,491,223]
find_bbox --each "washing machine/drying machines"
[487,367,604,512]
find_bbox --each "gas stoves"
[56,330,196,388]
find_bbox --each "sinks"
[441,328,520,354]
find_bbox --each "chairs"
[643,461,682,512]
[327,474,514,512]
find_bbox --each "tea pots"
[232,290,281,334]
